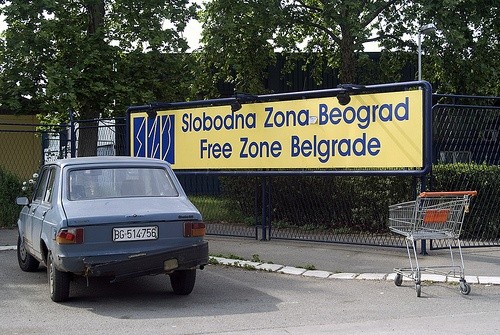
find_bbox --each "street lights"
[418,23,437,91]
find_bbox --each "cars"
[16,156,210,302]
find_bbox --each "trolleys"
[388,191,477,297]
